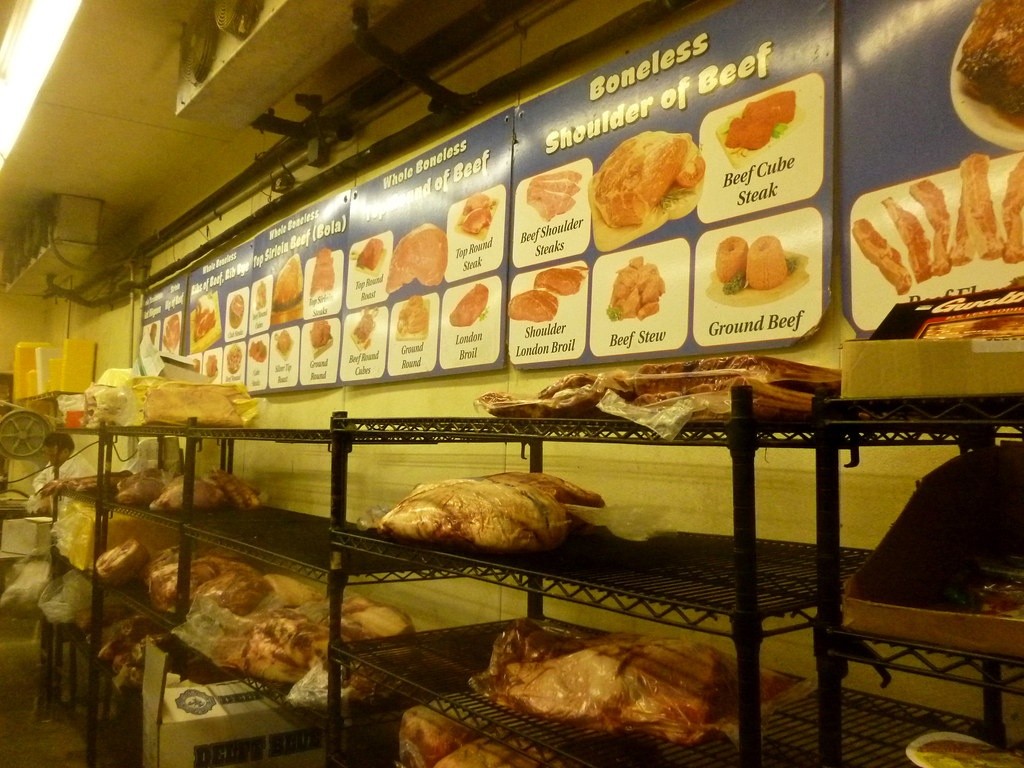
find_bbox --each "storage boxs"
[12,338,97,399]
[842,441,1024,661]
[1,516,59,554]
[142,634,349,768]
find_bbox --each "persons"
[34,433,95,515]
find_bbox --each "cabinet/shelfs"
[34,379,1024,768]
